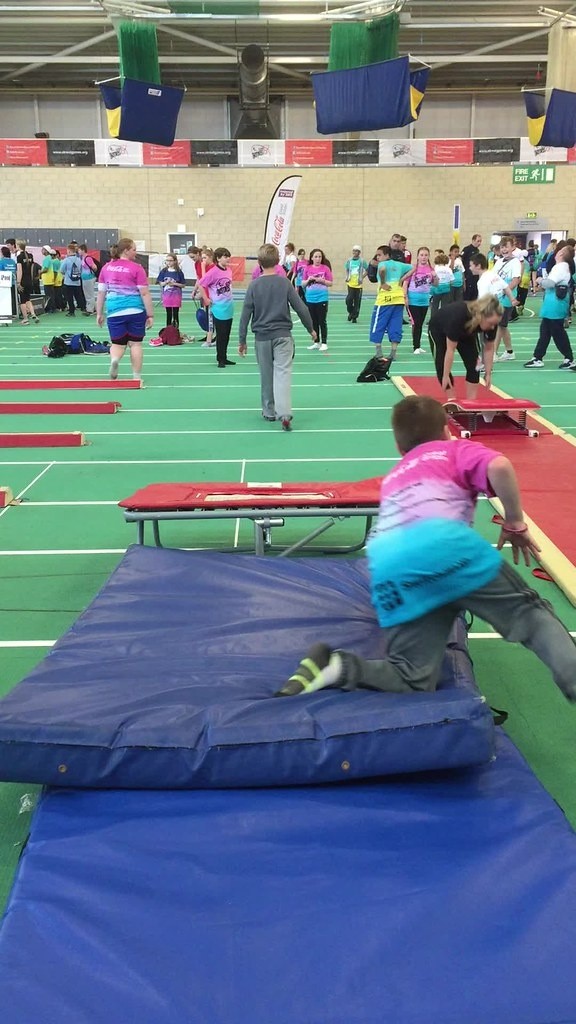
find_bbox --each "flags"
[264,175,302,268]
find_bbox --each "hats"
[353,246,361,254]
[43,245,51,253]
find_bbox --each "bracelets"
[503,523,528,534]
[147,315,153,319]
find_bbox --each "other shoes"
[82,310,90,316]
[31,315,39,323]
[414,349,420,354]
[262,411,276,421]
[419,348,426,353]
[19,321,28,325]
[352,317,356,323]
[65,311,74,317]
[319,344,327,350]
[282,417,292,431]
[307,342,318,349]
[201,341,212,347]
[508,316,519,323]
[348,312,354,320]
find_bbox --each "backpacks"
[68,258,80,281]
[83,256,102,278]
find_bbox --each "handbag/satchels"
[556,285,568,298]
[357,355,392,382]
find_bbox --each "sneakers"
[524,358,544,367]
[559,358,574,368]
[498,351,515,360]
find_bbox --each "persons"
[197,248,236,368]
[97,238,154,384]
[426,234,576,325]
[429,294,503,401]
[493,237,522,361]
[524,246,575,369]
[469,253,520,372]
[252,264,286,280]
[370,246,416,360]
[278,395,576,707]
[156,252,187,328]
[371,233,438,353]
[0,238,97,324]
[186,245,217,347]
[239,244,317,431]
[344,245,368,323]
[285,243,333,350]
